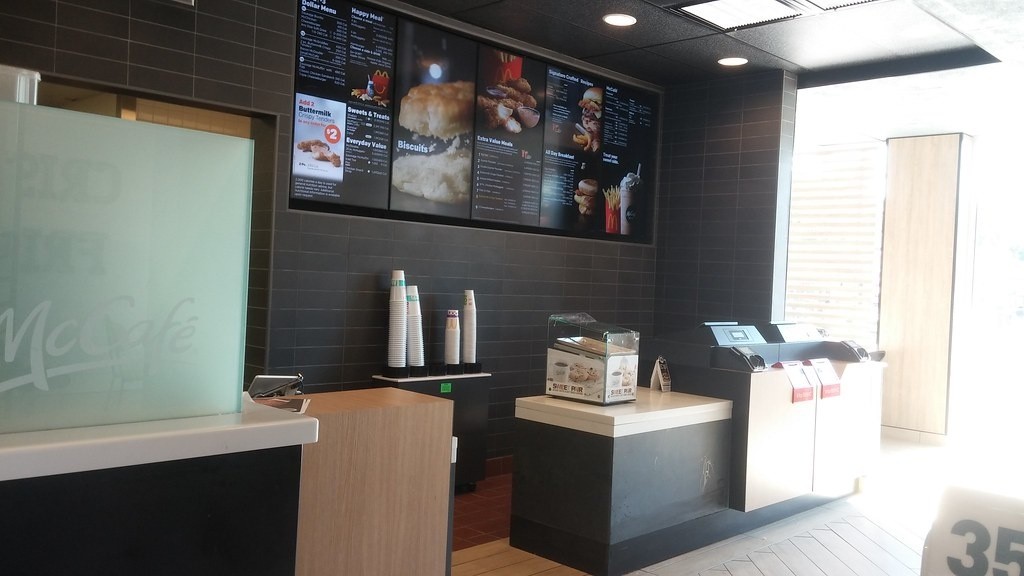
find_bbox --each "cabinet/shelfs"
[372,372,492,491]
[249,386,455,576]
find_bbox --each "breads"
[395,80,476,140]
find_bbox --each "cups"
[620,190,643,236]
[462,289,476,363]
[444,309,460,364]
[387,270,424,367]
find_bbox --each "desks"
[508,386,734,576]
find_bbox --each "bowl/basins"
[518,107,540,128]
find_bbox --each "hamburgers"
[578,86,603,151]
[575,179,599,215]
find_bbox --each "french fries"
[602,185,621,211]
[496,51,516,63]
